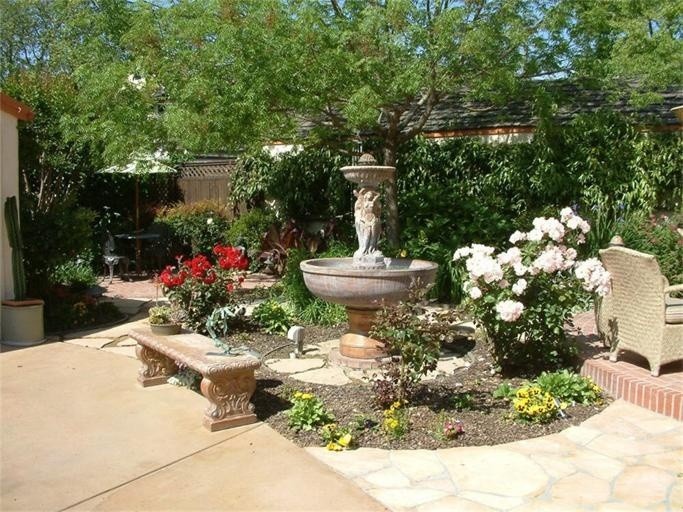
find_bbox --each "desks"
[113,233,158,277]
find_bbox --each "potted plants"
[147,305,180,332]
[0,196,48,347]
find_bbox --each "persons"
[353,187,381,255]
[94,147,178,277]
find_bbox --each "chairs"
[598,246,683,377]
[94,225,132,284]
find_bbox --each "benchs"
[128,326,261,432]
